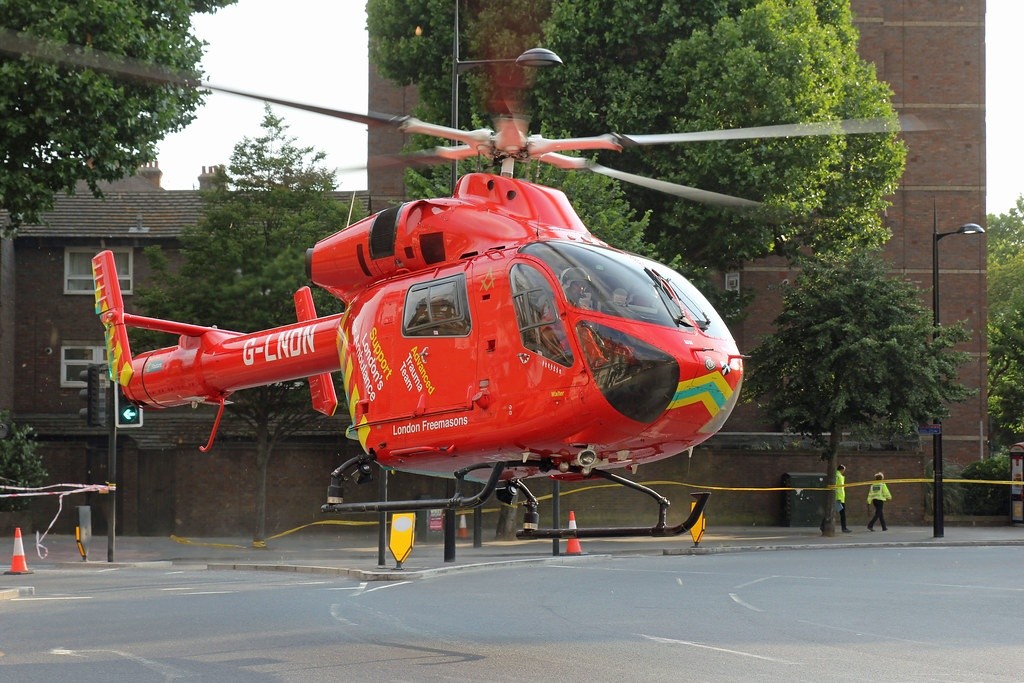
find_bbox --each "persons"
[535,269,616,362]
[820,465,853,533]
[866,472,892,533]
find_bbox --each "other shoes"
[867,525,875,531]
[843,528,852,533]
[882,528,888,531]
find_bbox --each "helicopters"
[0,37,942,541]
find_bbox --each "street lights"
[930,191,985,541]
[440,0,566,563]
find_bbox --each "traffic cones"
[558,511,592,556]
[4,528,33,575]
[458,514,471,540]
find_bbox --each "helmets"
[560,266,591,290]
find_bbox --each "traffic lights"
[112,374,144,429]
[78,365,107,429]
